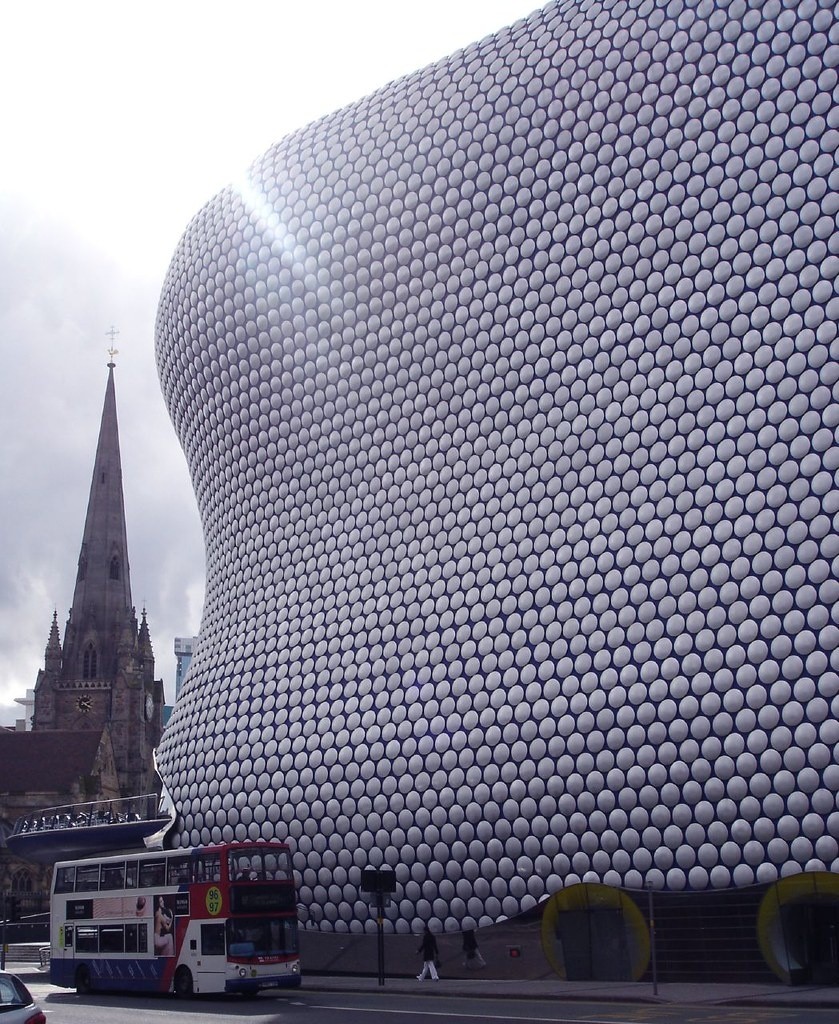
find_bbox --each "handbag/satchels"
[435,958,441,969]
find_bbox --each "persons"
[154,896,174,955]
[416,927,439,983]
[462,931,487,970]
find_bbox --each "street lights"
[2,896,16,971]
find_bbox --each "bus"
[48,841,315,997]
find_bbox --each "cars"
[0,971,47,1024]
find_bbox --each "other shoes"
[432,978,439,983]
[416,976,423,984]
